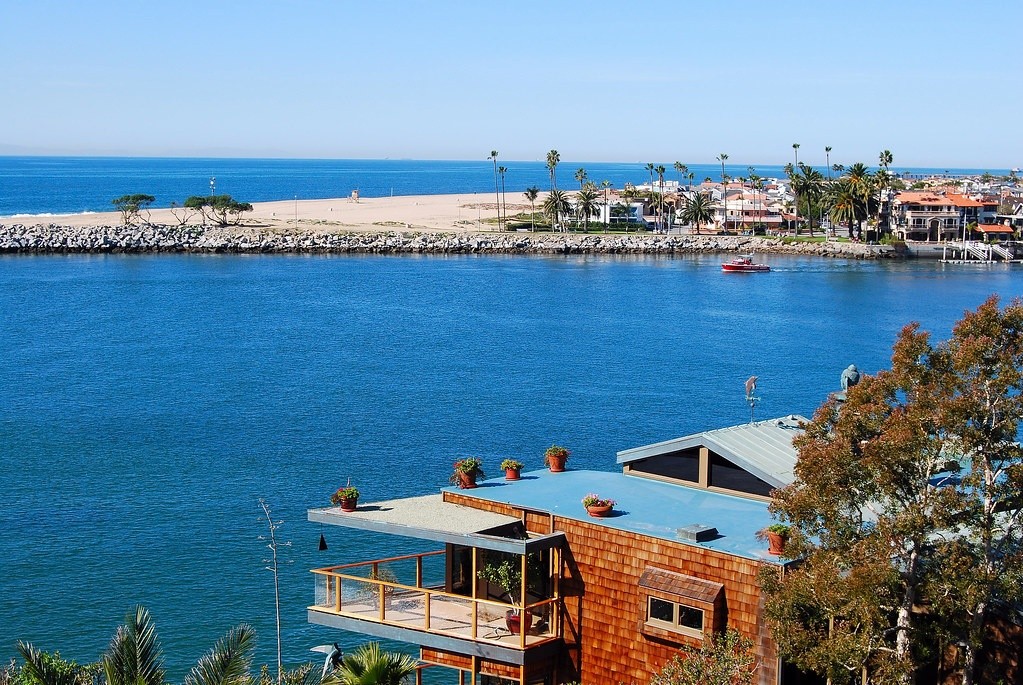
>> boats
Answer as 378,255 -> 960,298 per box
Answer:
721,256 -> 770,273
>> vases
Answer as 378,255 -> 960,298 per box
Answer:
459,471 -> 477,488
506,468 -> 520,479
767,532 -> 789,551
548,456 -> 566,469
588,504 -> 615,516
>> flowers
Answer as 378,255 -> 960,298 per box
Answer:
447,454 -> 487,489
754,523 -> 803,544
542,444 -> 571,466
500,458 -> 525,471
580,493 -> 618,512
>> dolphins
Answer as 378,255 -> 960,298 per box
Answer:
743,376 -> 758,398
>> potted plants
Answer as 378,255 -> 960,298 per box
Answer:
330,480 -> 361,513
364,570 -> 401,612
477,526 -> 543,634
